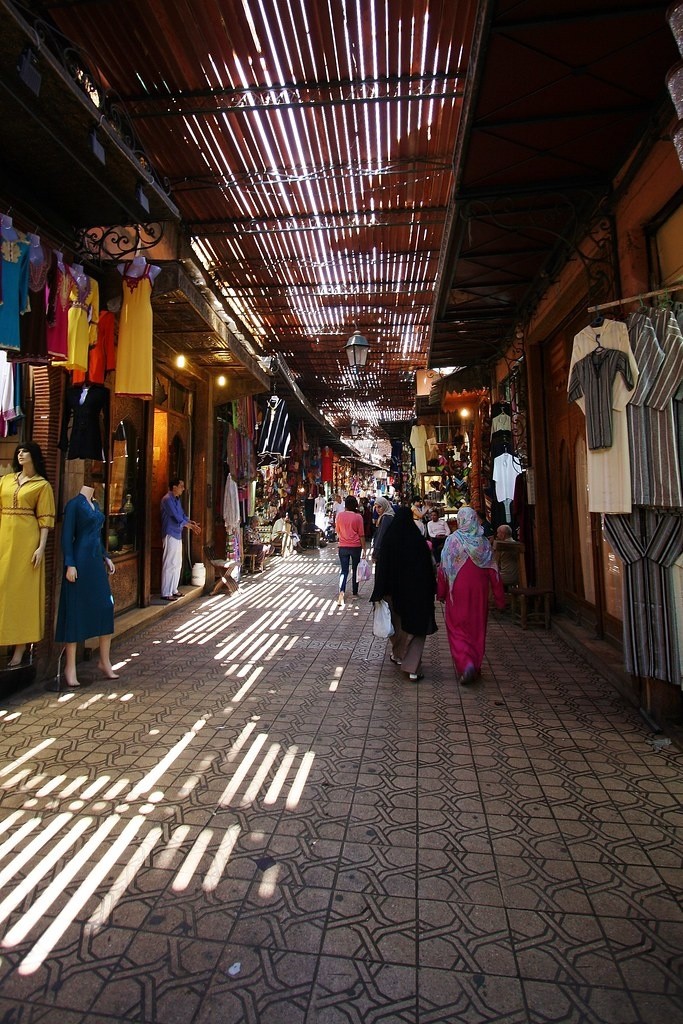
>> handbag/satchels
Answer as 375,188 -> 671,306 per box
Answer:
357,559 -> 371,583
371,600 -> 395,638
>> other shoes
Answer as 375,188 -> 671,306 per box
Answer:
337,592 -> 345,606
353,595 -> 362,600
459,666 -> 475,685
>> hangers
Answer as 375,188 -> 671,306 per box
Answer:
494,286 -> 672,457
268,384 -> 279,405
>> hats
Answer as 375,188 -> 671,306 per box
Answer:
413,495 -> 422,502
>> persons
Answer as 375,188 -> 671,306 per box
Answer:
436,506 -> 505,685
306,492 -> 326,531
428,510 -> 451,567
271,509 -> 307,556
160,478 -> 201,601
0,440 -> 57,669
372,497 -> 395,565
371,506 -> 438,680
488,524 -> 524,610
334,495 -> 366,606
54,484 -> 119,686
244,515 -> 270,572
476,509 -> 494,545
339,491 -> 437,542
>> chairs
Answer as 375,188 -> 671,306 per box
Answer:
203,545 -> 240,597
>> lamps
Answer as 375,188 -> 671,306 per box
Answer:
342,293 -> 371,367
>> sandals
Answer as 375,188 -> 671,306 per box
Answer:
173,592 -> 186,597
389,652 -> 402,665
162,596 -> 177,601
409,673 -> 426,682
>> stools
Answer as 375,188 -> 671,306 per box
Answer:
244,552 -> 264,573
509,587 -> 554,629
270,543 -> 283,556
